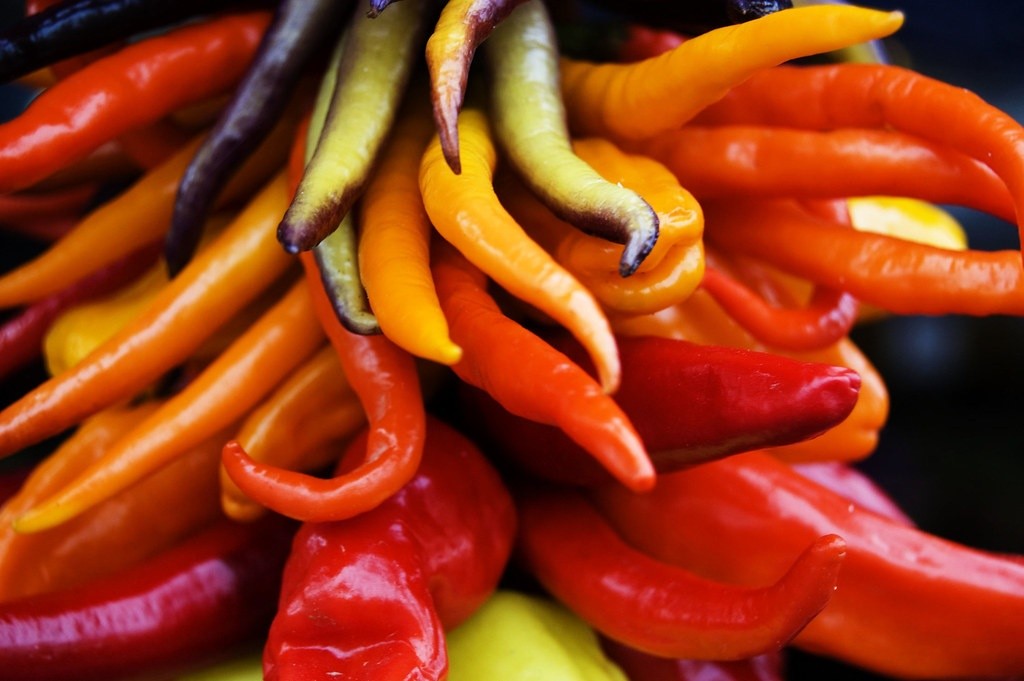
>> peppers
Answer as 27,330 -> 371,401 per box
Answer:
0,0 -> 1024,681
